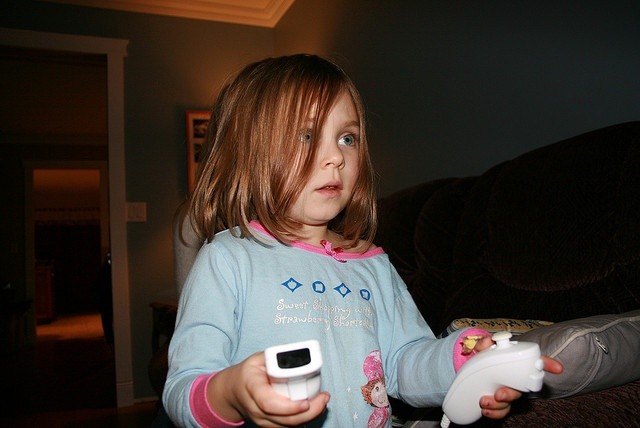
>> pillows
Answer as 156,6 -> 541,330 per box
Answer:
437,317 -> 554,364
512,309 -> 640,400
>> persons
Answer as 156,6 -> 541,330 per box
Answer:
162,53 -> 564,428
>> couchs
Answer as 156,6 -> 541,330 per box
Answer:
71,121 -> 640,428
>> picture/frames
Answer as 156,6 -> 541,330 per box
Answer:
185,109 -> 213,195
